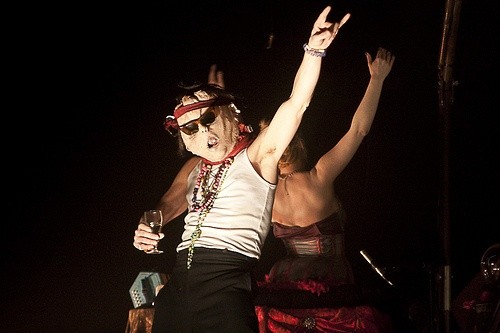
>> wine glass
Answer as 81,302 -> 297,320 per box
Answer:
143,211 -> 164,255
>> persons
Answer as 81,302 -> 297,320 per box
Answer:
256,48 -> 395,333
133,6 -> 351,333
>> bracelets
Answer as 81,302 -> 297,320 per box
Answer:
303,43 -> 326,57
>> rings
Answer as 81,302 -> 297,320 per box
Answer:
138,243 -> 142,250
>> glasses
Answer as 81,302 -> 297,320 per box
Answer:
178,107 -> 216,135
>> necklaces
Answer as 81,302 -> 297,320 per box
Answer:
187,156 -> 234,268
278,170 -> 297,195
